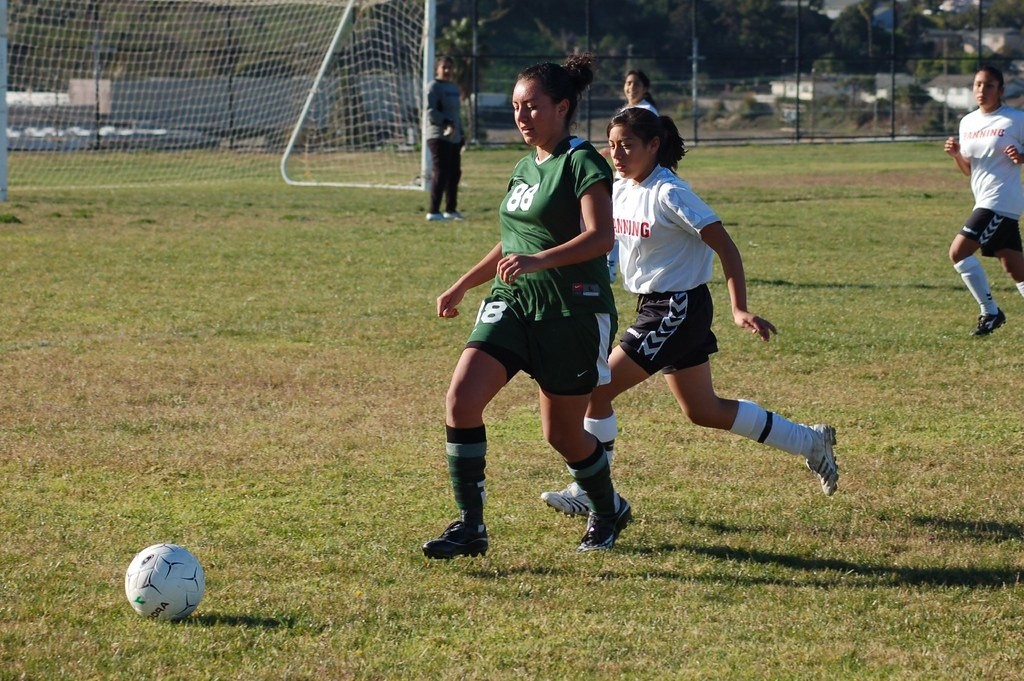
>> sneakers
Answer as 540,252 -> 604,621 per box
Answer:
422,521 -> 489,560
540,482 -> 592,518
576,494 -> 633,553
804,424 -> 839,496
969,307 -> 1006,336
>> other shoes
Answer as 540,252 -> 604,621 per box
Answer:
426,213 -> 447,220
608,265 -> 616,283
441,211 -> 463,219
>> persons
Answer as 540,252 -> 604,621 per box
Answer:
426,55 -> 466,220
540,106 -> 839,517
606,68 -> 659,283
421,53 -> 633,560
945,67 -> 1024,335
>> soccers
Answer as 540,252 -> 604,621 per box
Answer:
124,542 -> 205,622
